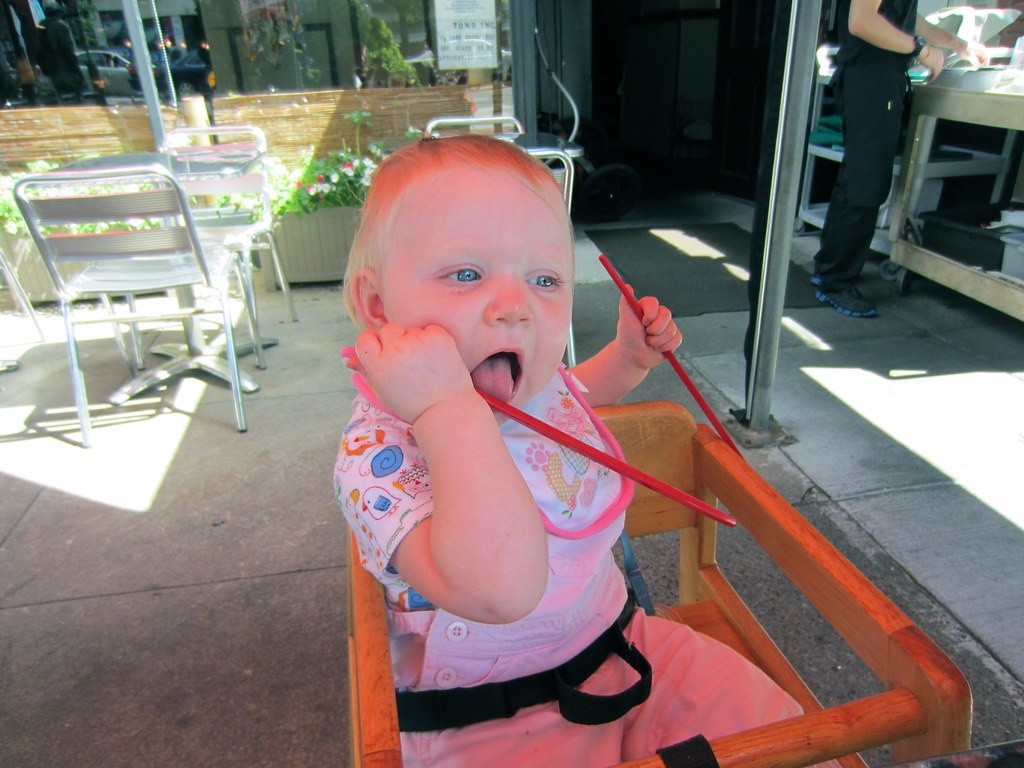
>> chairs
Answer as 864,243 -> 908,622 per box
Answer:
423,115 -> 577,371
14,165 -> 270,451
340,401 -> 975,768
160,123 -> 298,369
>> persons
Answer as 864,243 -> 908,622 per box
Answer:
805,0 -> 990,320
332,133 -> 803,767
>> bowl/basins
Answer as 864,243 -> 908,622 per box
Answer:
938,70 -> 1002,91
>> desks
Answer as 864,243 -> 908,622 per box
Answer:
25,142 -> 269,374
372,131 -> 584,160
794,76 -> 1024,320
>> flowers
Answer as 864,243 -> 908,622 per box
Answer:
0,75 -> 442,239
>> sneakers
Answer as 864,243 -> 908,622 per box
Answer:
810,268 -> 825,286
816,284 -> 877,317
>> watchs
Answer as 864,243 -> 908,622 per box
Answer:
909,34 -> 925,57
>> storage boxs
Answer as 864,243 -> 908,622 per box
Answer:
0,206 -> 363,314
918,200 -> 1024,271
928,68 -> 1005,91
999,233 -> 1024,281
